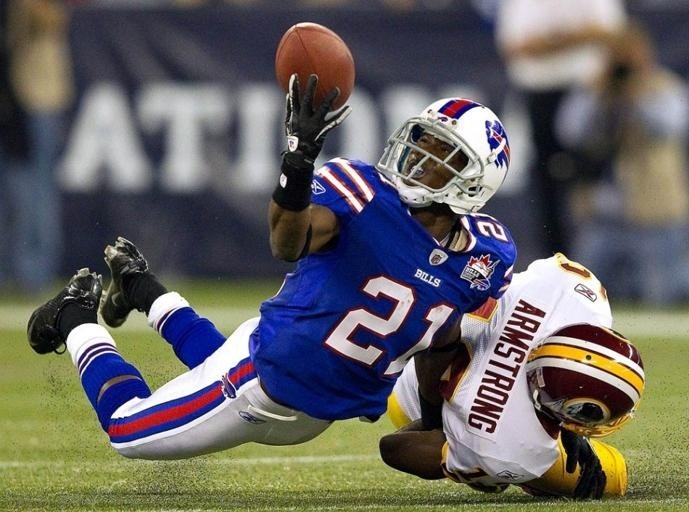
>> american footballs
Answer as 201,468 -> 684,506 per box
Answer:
275,23 -> 355,111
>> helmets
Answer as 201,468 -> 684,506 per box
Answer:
375,96 -> 511,218
525,322 -> 646,439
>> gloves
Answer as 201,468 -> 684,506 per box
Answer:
560,425 -> 608,502
270,72 -> 352,213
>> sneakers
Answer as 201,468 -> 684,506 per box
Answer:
26,267 -> 104,355
100,237 -> 150,329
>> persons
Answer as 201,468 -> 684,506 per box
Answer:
552,17 -> 689,313
493,0 -> 634,254
378,249 -> 648,500
1,0 -> 77,294
26,69 -> 520,463
